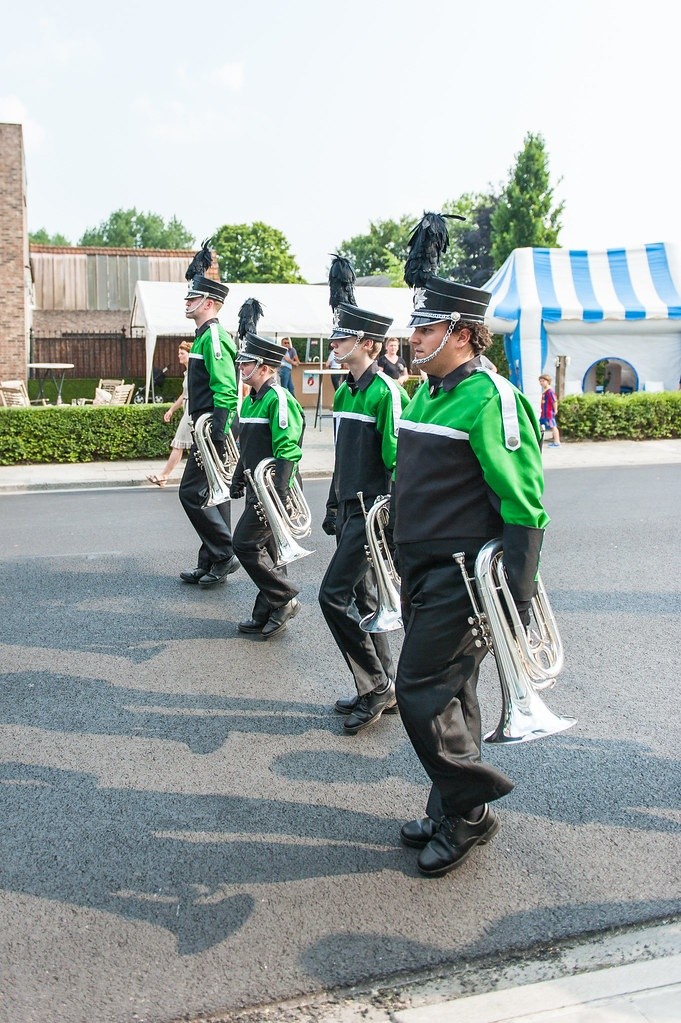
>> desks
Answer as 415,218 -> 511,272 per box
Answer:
28,362 -> 74,405
304,369 -> 351,432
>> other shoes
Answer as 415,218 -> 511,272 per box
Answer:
547,443 -> 560,448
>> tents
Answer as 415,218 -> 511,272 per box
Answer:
477,241 -> 681,431
129,280 -> 424,406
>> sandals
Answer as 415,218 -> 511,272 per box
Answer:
145,475 -> 168,487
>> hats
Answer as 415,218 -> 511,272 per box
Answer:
234,298 -> 288,367
328,254 -> 394,342
184,237 -> 229,305
404,210 -> 493,328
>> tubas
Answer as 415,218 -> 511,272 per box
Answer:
453,534 -> 579,747
243,457 -> 317,571
356,491 -> 405,634
186,411 -> 240,510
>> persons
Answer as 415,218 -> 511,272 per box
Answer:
318,301 -> 410,732
233,331 -> 305,638
178,276 -> 241,586
391,278 -> 549,877
144,338 -> 561,487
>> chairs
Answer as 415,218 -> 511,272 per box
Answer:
0,377 -> 135,409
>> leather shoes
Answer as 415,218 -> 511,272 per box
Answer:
238,597 -> 302,637
180,568 -> 227,584
344,678 -> 398,733
198,554 -> 242,585
401,802 -> 501,878
335,695 -> 398,714
